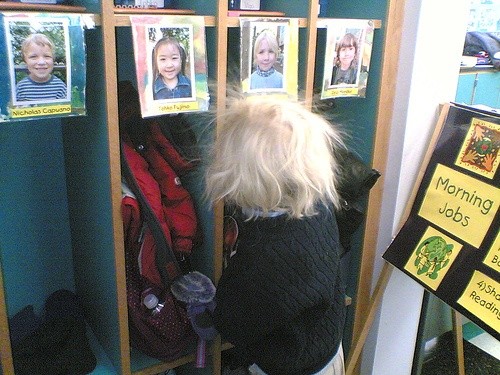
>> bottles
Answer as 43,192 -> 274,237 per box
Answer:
144,294 -> 166,324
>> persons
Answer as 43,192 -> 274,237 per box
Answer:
204,94 -> 347,375
16,34 -> 67,100
152,37 -> 191,100
332,34 -> 357,84
251,30 -> 283,88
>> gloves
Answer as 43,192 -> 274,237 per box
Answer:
191,298 -> 218,343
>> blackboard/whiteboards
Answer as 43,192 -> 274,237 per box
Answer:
379,100 -> 500,341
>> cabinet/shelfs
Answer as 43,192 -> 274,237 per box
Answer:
455,66 -> 500,113
0,0 -> 406,375
